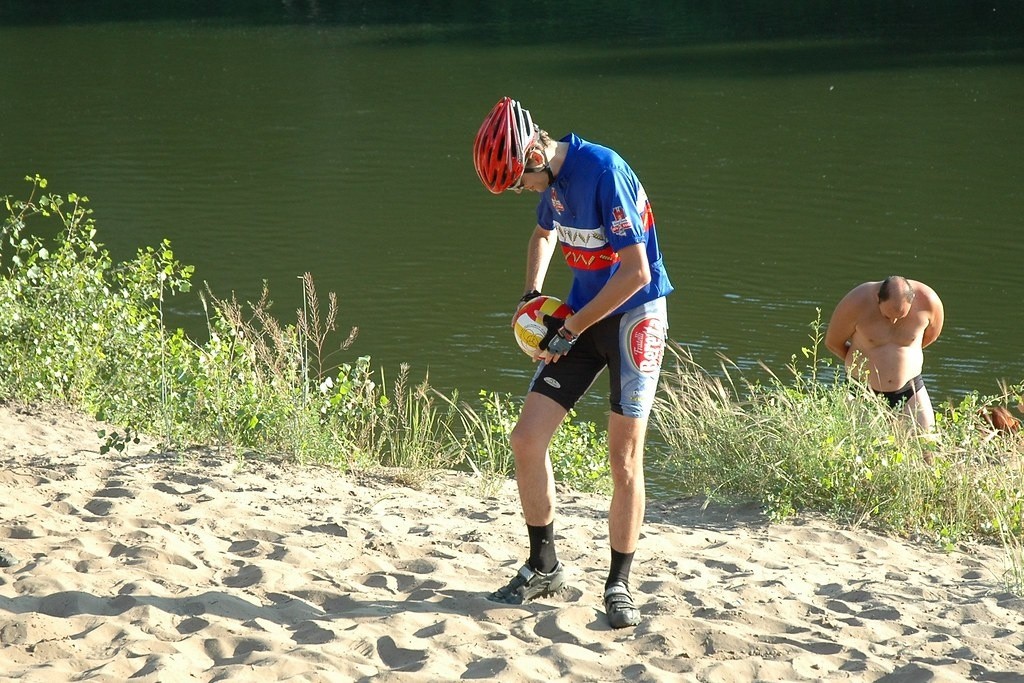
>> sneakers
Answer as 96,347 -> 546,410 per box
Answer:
603,580 -> 641,629
485,559 -> 564,604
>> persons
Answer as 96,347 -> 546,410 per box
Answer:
473,96 -> 674,628
825,276 -> 945,467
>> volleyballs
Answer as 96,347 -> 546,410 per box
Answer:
513,294 -> 578,362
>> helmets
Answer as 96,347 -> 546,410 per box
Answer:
472,95 -> 538,195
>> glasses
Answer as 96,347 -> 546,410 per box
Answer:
505,150 -> 534,191
